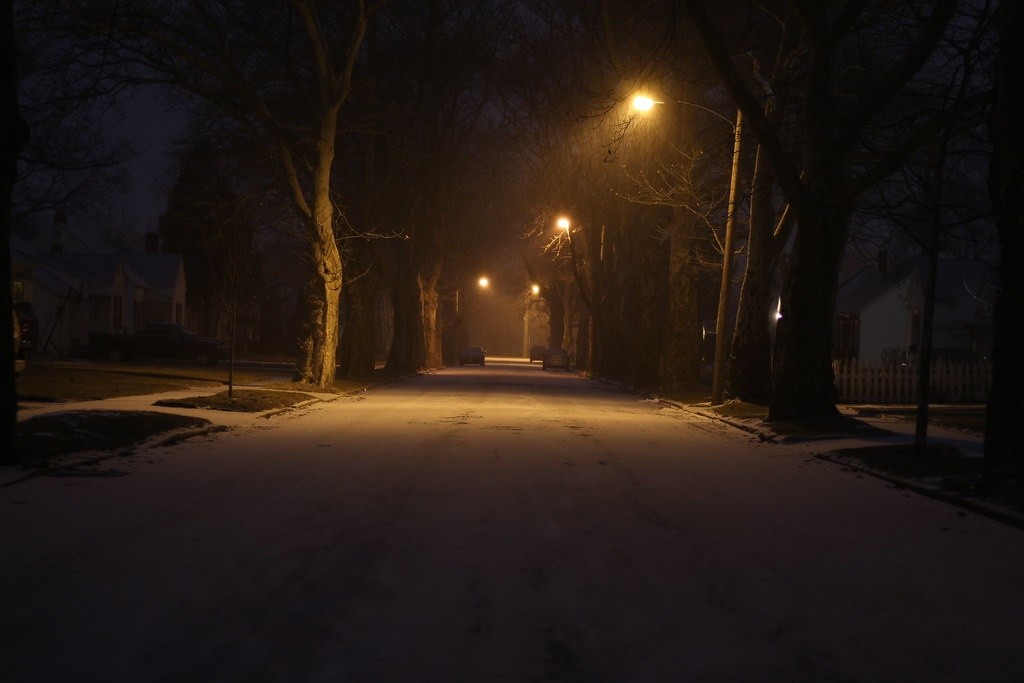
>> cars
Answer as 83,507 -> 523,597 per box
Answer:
457,344 -> 487,366
529,344 -> 546,364
542,347 -> 570,371
84,319 -> 225,366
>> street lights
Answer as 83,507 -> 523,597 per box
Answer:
632,93 -> 750,409
